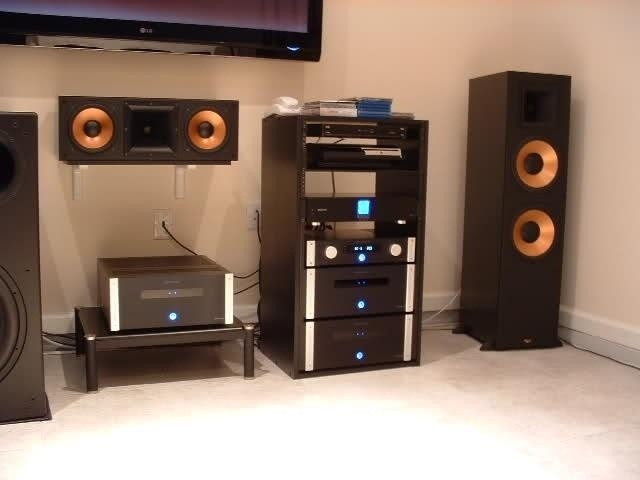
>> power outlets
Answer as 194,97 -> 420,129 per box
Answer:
152,209 -> 175,240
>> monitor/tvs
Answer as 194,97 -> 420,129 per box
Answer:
0,0 -> 323,61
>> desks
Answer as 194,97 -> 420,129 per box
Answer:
75,306 -> 255,391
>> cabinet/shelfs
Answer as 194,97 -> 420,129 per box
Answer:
262,114 -> 430,379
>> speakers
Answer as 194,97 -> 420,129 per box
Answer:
0,112 -> 52,425
59,96 -> 239,165
451,70 -> 571,351
